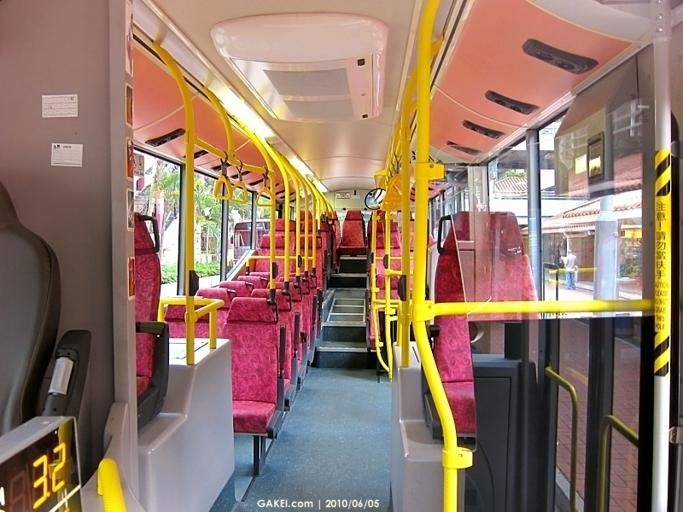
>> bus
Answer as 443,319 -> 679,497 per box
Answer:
231,219 -> 270,263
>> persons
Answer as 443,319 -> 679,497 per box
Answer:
565,250 -> 578,290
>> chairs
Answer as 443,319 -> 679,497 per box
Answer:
135,208 -> 549,478
2,185 -> 92,444
